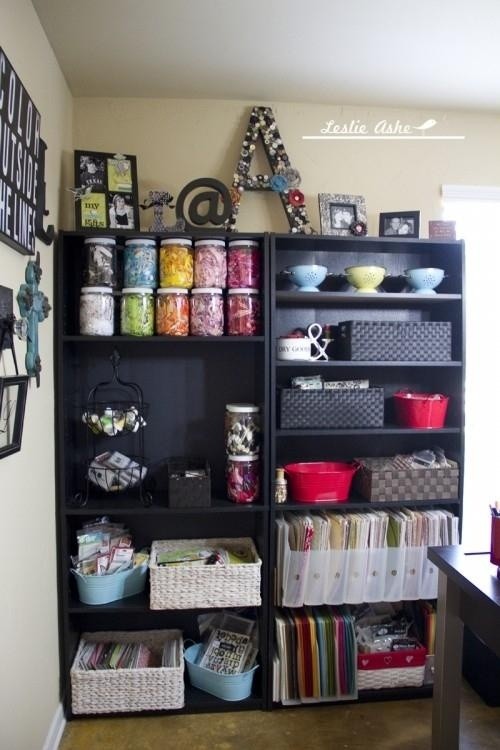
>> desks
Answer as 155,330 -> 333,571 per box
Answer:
428,545 -> 500,750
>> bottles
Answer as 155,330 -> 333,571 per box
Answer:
323,322 -> 332,339
275,468 -> 288,504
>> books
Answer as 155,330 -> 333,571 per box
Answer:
196,613 -> 258,677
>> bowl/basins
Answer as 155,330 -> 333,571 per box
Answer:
284,461 -> 362,503
393,388 -> 449,430
279,263 -> 334,290
339,264 -> 393,291
397,265 -> 452,292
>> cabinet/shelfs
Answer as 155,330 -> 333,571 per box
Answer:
269,234 -> 469,707
55,228 -> 268,718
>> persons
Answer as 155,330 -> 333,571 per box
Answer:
384,217 -> 402,235
398,222 -> 412,235
333,207 -> 345,230
342,207 -> 355,229
108,193 -> 136,230
80,158 -> 106,190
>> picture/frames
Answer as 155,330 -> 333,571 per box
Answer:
318,192 -> 367,236
379,210 -> 420,238
74,149 -> 140,234
0,374 -> 30,459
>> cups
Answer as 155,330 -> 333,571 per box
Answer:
274,323 -> 332,361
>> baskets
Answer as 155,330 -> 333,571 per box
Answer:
338,320 -> 453,361
81,402 -> 150,441
81,455 -> 154,499
149,537 -> 262,611
358,664 -> 426,690
357,456 -> 459,504
70,629 -> 186,715
280,385 -> 386,430
